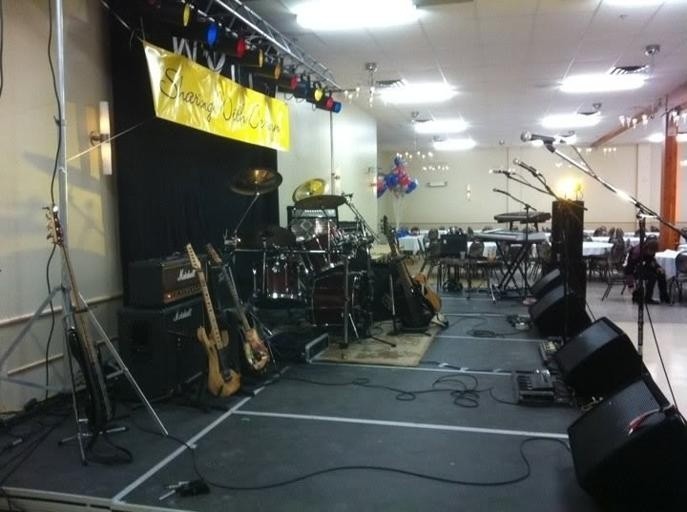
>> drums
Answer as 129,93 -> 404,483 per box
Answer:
313,272 -> 363,336
295,234 -> 345,278
251,258 -> 299,309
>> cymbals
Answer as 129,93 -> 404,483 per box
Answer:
247,225 -> 295,245
292,179 -> 325,203
224,166 -> 282,196
294,195 -> 348,210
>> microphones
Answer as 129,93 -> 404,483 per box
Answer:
489,168 -> 511,176
513,158 -> 539,176
493,187 -> 514,196
520,131 -> 567,144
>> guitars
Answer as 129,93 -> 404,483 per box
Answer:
186,243 -> 241,397
204,243 -> 270,372
380,215 -> 441,327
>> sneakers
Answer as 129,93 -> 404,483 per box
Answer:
645,297 -> 670,304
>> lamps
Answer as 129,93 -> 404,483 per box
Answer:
145,0 -> 341,112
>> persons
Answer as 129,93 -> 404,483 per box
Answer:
626,233 -> 671,305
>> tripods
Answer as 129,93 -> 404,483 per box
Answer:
241,203 -> 394,347
0,1 -> 169,462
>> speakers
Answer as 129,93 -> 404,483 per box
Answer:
566,374 -> 687,512
528,266 -> 560,297
117,295 -> 205,397
551,200 -> 584,298
553,316 -> 646,389
528,282 -> 586,336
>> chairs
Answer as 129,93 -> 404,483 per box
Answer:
395,227 -> 687,306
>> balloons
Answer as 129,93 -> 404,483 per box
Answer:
376,154 -> 419,200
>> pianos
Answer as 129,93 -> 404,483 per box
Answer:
472,228 -> 545,242
495,212 -> 551,223
